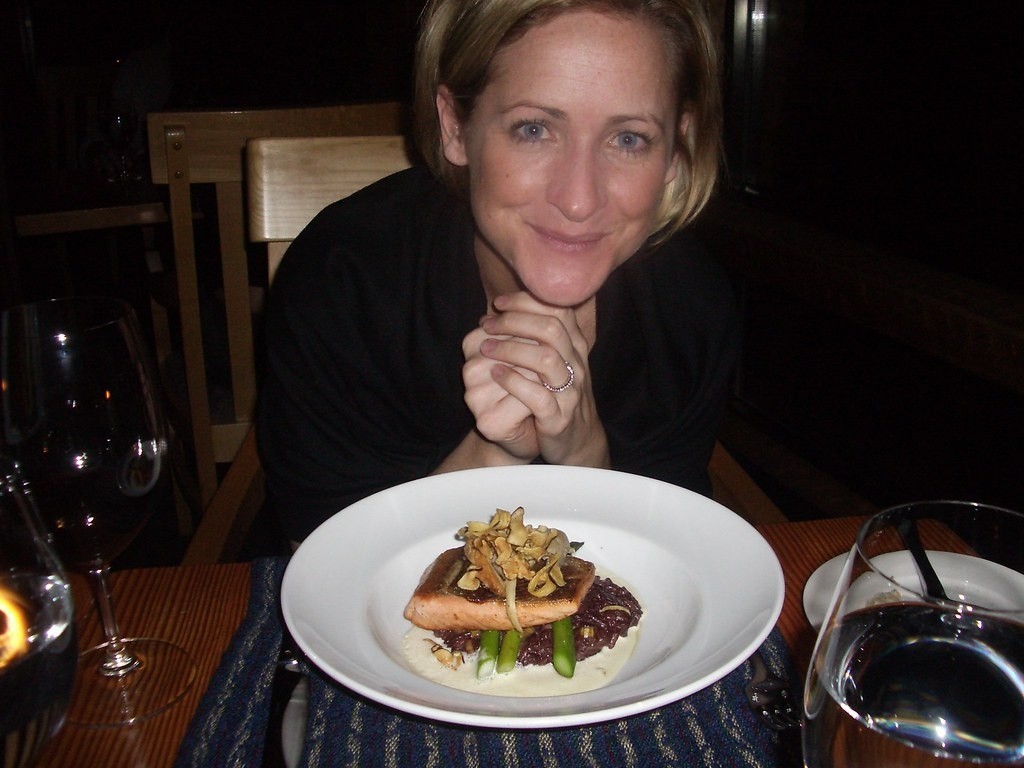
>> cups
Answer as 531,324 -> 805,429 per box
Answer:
0,461 -> 86,768
800,498 -> 1024,768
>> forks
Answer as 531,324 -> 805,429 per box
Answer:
742,650 -> 808,731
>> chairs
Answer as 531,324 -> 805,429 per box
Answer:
149,101 -> 421,512
180,136 -> 789,566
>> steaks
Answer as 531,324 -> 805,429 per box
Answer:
404,546 -> 595,629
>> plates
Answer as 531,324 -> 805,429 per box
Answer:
280,464 -> 786,730
803,549 -> 1024,636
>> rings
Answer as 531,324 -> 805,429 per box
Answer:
543,362 -> 574,392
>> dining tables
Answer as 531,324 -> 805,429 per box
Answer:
0,515 -> 978,768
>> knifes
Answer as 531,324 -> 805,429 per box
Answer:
282,677 -> 309,768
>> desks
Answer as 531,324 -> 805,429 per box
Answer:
12,188 -> 208,538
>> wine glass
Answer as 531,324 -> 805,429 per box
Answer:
0,298 -> 200,730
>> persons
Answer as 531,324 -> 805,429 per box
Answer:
251,0 -> 736,556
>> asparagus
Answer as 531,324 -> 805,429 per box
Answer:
478,633 -> 496,678
553,619 -> 576,677
499,632 -> 520,671
